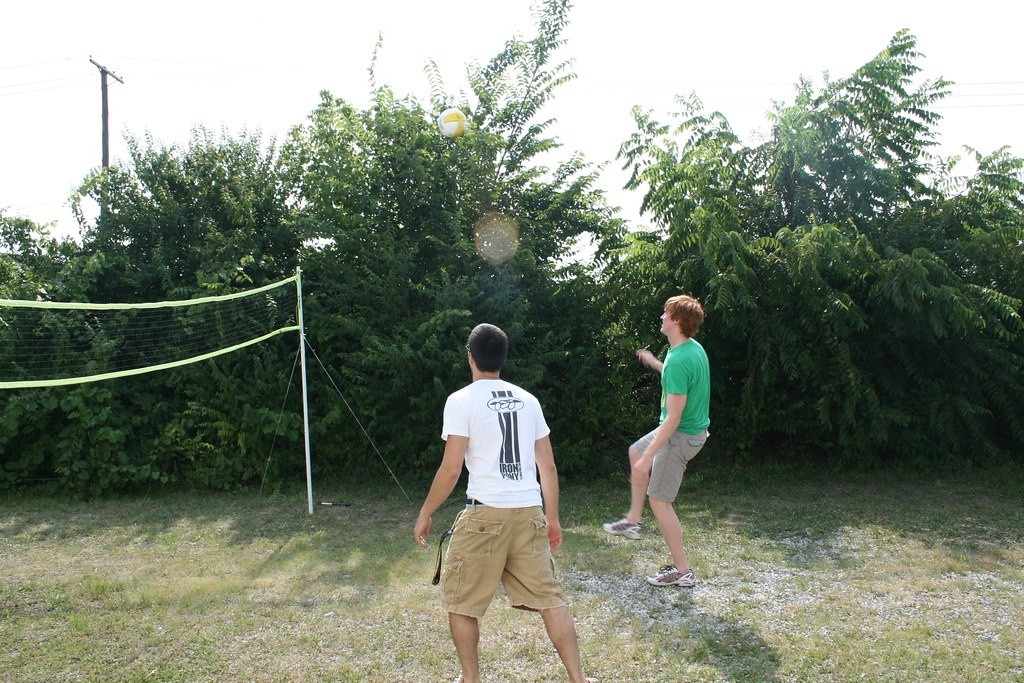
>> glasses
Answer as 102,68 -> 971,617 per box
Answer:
465,344 -> 469,351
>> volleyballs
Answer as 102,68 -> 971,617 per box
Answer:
438,108 -> 468,138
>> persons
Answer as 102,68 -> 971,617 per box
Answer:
602,295 -> 711,586
416,324 -> 602,683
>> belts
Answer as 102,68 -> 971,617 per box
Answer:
466,499 -> 483,506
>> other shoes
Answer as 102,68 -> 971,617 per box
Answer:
453,669 -> 463,683
585,677 -> 599,683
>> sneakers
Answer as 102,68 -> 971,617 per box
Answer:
647,565 -> 696,586
604,519 -> 644,540
